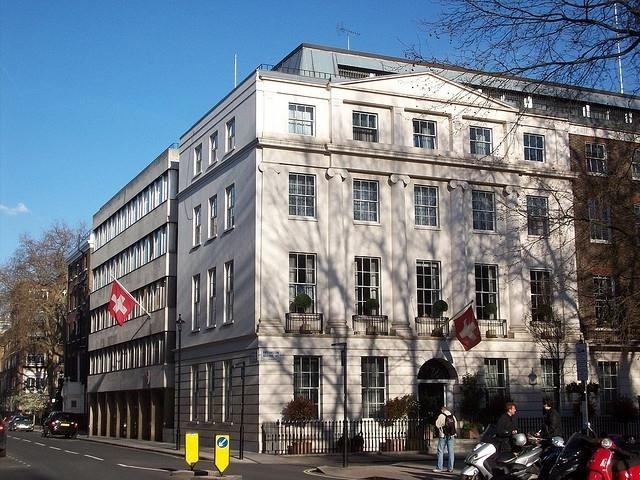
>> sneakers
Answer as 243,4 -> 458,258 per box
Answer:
432,469 -> 454,473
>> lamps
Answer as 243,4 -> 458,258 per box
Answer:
528,367 -> 538,390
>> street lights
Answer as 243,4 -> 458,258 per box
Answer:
174,314 -> 186,450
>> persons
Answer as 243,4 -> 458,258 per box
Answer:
535,397 -> 563,438
495,402 -> 519,453
432,405 -> 458,472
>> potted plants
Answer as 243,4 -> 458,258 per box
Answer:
432,299 -> 448,338
295,294 -> 313,334
538,306 -> 554,339
462,423 -> 482,439
338,434 -> 364,454
279,397 -> 315,454
371,394 -> 422,453
364,298 -> 379,334
485,303 -> 497,338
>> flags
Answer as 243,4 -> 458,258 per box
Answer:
454,305 -> 481,351
108,280 -> 136,326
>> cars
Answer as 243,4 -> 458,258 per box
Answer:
0,415 -> 7,457
9,415 -> 34,432
42,411 -> 79,438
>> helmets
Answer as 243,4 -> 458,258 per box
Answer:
511,432 -> 528,446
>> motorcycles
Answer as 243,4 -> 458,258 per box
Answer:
462,422 -> 640,479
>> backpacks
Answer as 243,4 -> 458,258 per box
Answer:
439,412 -> 457,437
